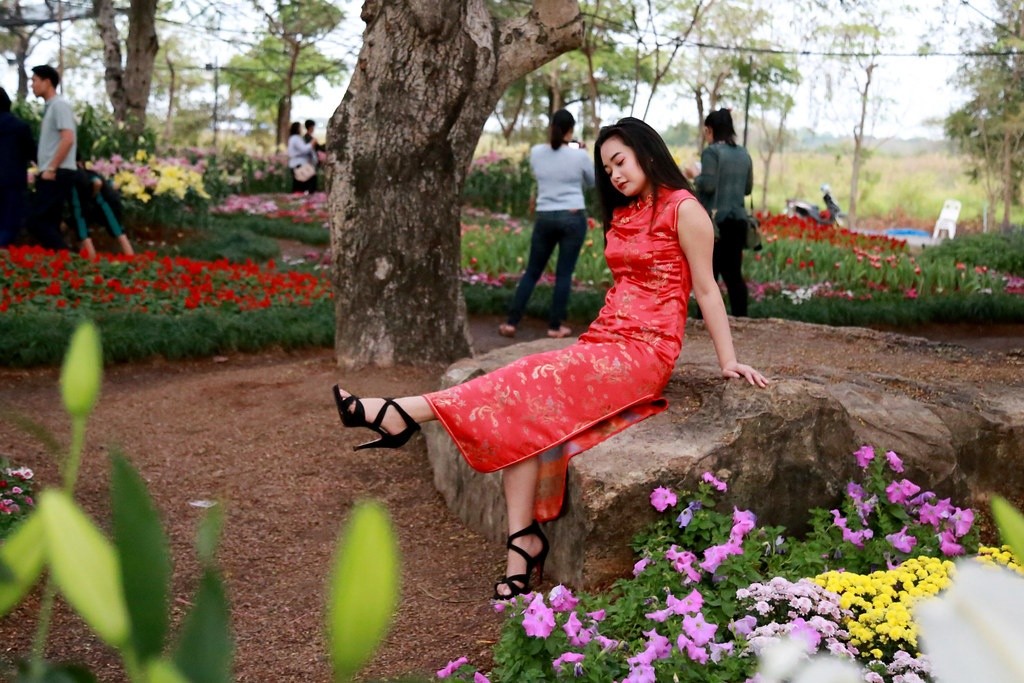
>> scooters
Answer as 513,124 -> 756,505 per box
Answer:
783,185 -> 850,232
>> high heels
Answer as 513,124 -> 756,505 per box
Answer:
332,383 -> 422,451
493,519 -> 549,601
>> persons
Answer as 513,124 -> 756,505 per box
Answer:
497,109 -> 595,339
287,119 -> 326,195
332,117 -> 771,600
0,65 -> 135,261
684,107 -> 754,320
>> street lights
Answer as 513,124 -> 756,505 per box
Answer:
205,57 -> 219,148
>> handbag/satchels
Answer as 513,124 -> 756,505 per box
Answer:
711,217 -> 719,242
294,161 -> 317,182
745,215 -> 762,251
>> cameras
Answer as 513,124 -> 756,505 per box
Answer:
568,141 -> 582,150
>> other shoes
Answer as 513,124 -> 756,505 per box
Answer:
548,327 -> 572,338
498,323 -> 515,336
